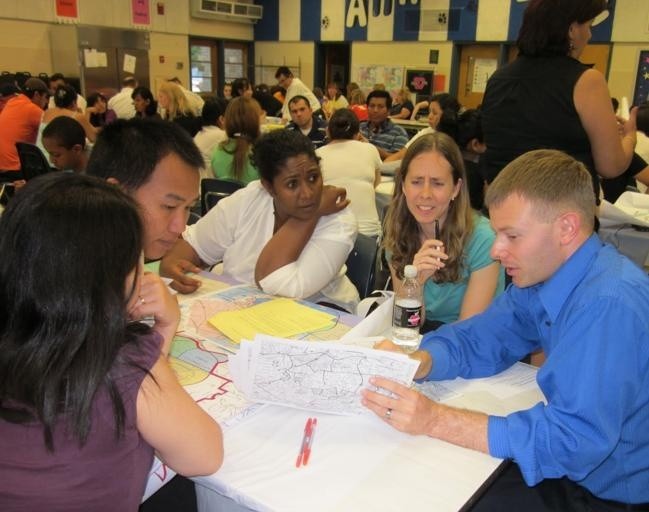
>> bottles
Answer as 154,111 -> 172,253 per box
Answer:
390,265 -> 420,349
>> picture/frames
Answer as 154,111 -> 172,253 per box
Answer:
420,373 -> 422,375
244,335 -> 419,416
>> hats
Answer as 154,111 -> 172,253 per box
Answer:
24,78 -> 53,96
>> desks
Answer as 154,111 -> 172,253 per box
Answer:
390,116 -> 430,134
375,172 -> 396,211
142,252 -> 546,512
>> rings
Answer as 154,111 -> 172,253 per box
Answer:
139,295 -> 145,304
385,409 -> 392,419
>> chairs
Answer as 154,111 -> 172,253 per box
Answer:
200,177 -> 247,218
0,141 -> 55,179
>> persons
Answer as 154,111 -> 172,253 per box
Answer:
388,87 -> 413,119
159,82 -> 196,135
192,97 -> 229,202
48,72 -> 88,118
438,110 -> 487,212
81,115 -> 206,261
359,148 -> 649,512
379,131 -> 506,323
231,77 -> 252,99
157,128 -> 360,315
346,83 -> 361,104
0,76 -> 52,184
275,66 -> 322,127
223,82 -> 231,97
0,172 -> 224,512
601,148 -> 649,204
349,89 -> 368,123
107,76 -> 139,120
35,84 -> 101,171
0,78 -> 17,111
86,92 -> 116,128
283,95 -> 329,150
323,82 -> 349,115
357,90 -> 409,160
482,0 -> 639,220
382,92 -> 461,163
314,106 -> 383,301
40,115 -> 95,177
131,86 -> 161,121
410,94 -> 431,121
207,96 -> 263,187
312,86 -> 325,108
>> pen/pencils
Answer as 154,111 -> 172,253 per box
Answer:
296,417 -> 311,468
302,417 -> 318,467
434,219 -> 442,268
